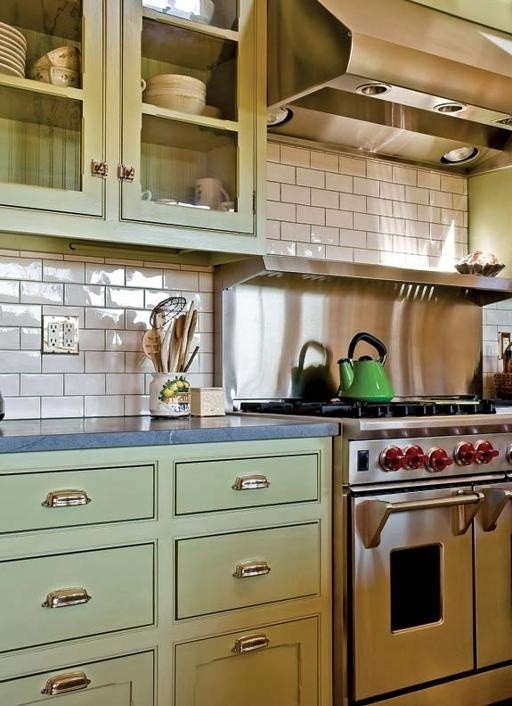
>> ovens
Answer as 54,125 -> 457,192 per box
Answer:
333,473 -> 512,706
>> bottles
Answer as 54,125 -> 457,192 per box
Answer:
148,371 -> 192,417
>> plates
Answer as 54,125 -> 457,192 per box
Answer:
454,262 -> 505,277
0,21 -> 28,78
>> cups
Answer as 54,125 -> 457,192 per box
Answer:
194,176 -> 231,210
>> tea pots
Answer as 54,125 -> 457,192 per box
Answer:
289,338 -> 337,401
335,333 -> 394,403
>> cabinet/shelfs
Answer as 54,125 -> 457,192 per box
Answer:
0,433 -> 339,706
0,0 -> 274,265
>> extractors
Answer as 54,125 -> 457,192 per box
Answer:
205,0 -> 511,178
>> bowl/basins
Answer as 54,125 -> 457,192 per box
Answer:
203,104 -> 224,118
31,45 -> 82,88
145,74 -> 208,117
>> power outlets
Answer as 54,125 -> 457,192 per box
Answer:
42,314 -> 81,355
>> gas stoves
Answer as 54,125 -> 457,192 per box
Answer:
234,397 -> 511,485
